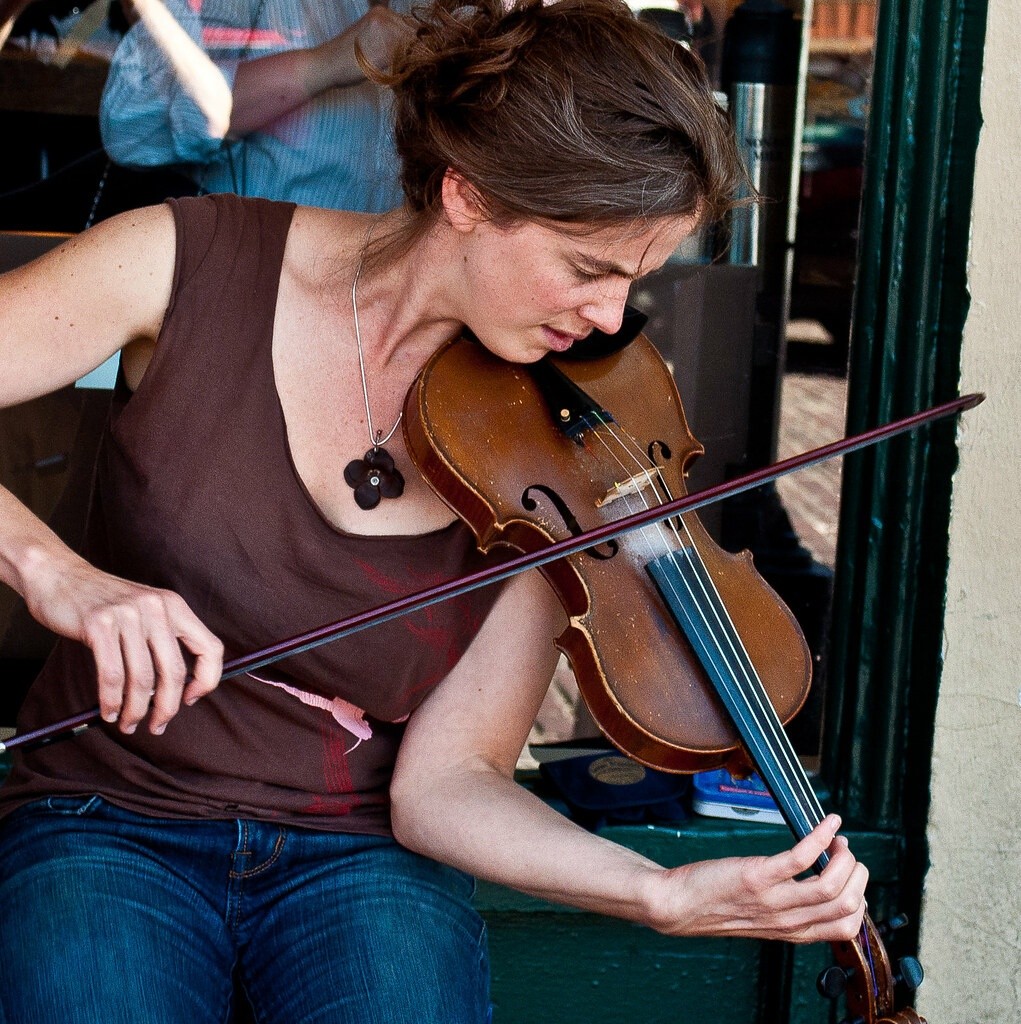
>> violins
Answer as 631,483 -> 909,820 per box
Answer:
401,319 -> 929,1024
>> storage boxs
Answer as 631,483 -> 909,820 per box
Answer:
690,768 -> 787,826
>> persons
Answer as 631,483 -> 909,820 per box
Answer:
101,0 -> 441,218
2,2 -> 871,1023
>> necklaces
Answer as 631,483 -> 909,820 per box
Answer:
343,206 -> 438,512
